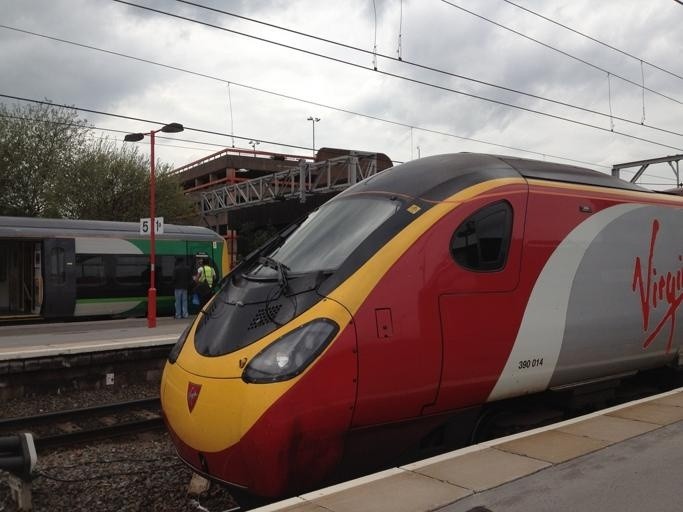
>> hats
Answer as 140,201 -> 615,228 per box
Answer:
195,252 -> 209,258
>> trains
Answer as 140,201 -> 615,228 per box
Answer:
0,216 -> 230,329
155,151 -> 682,506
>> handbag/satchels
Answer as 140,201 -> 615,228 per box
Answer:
194,279 -> 212,300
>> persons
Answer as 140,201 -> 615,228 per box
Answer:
171,251 -> 219,319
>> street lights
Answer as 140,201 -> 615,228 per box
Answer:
306,116 -> 320,161
248,141 -> 260,157
123,123 -> 185,330
